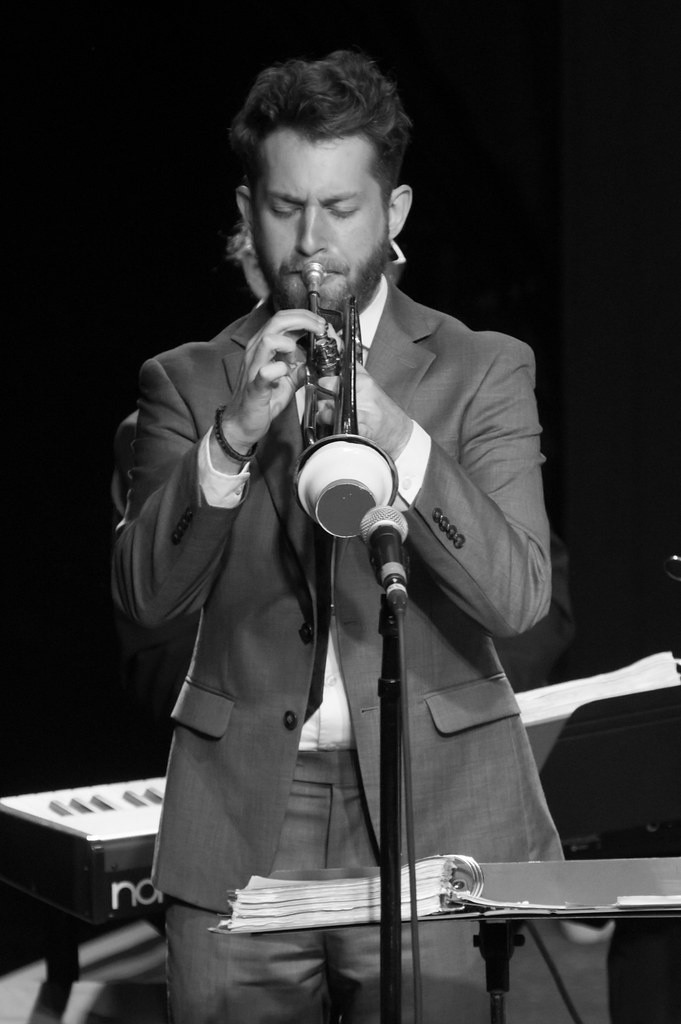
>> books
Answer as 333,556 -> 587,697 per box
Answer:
210,854 -> 681,935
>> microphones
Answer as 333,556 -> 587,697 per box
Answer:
360,505 -> 409,610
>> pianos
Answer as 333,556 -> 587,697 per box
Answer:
1,775 -> 170,923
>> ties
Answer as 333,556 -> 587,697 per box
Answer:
303,349 -> 333,725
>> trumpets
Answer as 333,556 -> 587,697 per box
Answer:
292,261 -> 401,539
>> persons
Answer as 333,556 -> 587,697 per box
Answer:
114,54 -> 568,1024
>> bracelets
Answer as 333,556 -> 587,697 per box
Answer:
215,405 -> 256,461
